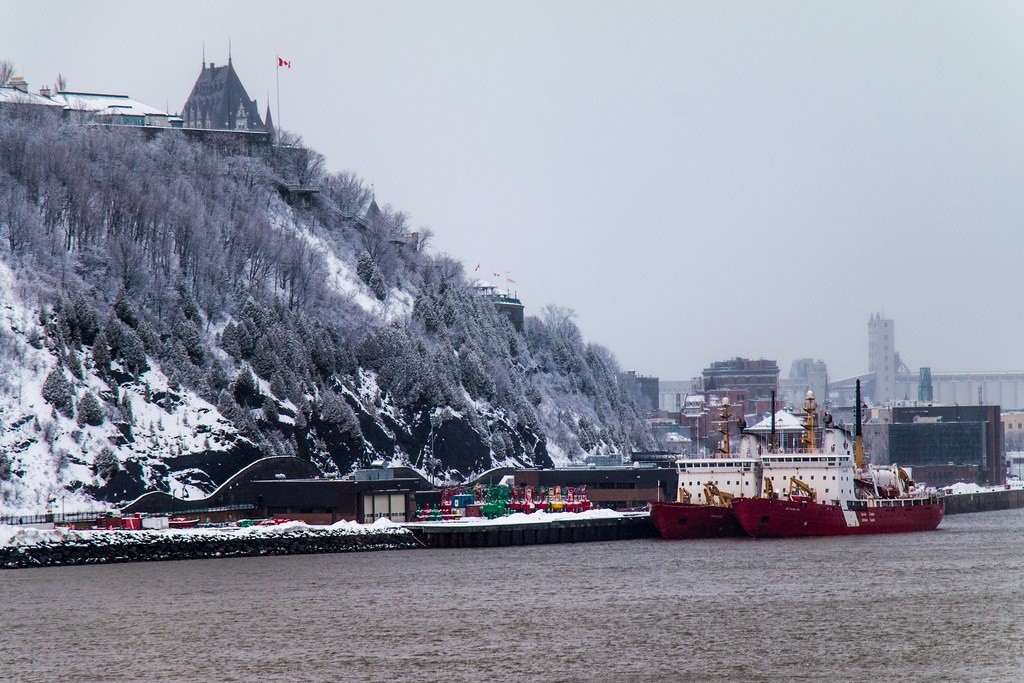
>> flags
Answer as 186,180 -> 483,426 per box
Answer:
278,57 -> 291,69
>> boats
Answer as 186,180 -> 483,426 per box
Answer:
646,452 -> 947,539
